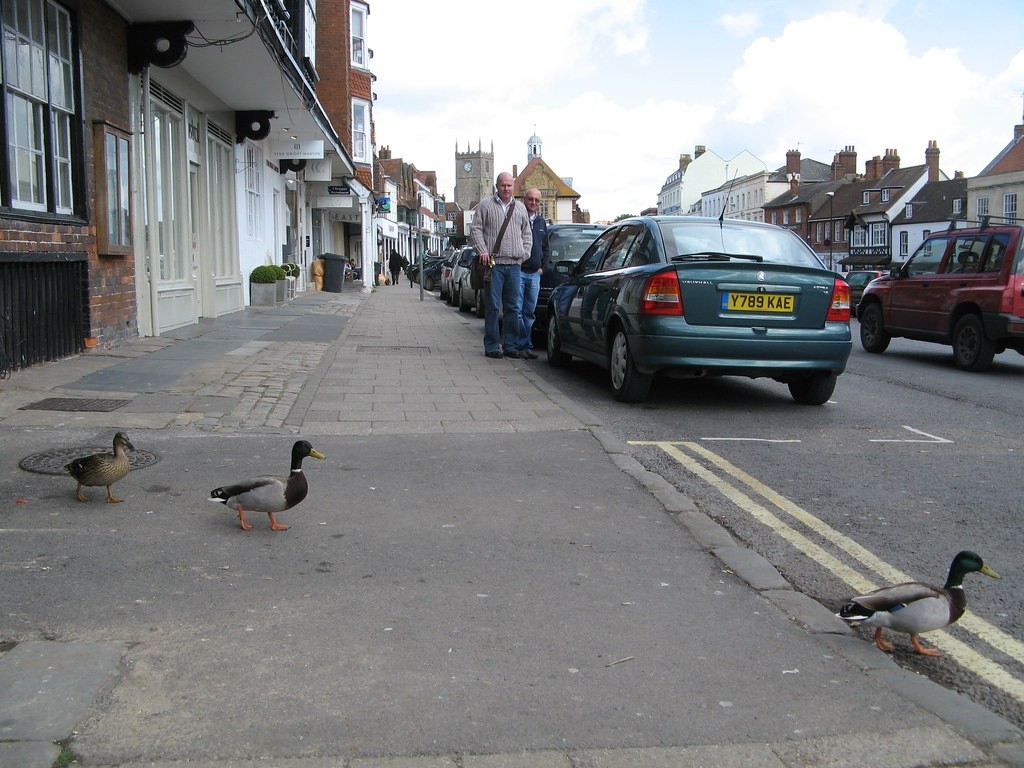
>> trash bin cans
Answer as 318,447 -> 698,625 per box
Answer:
374,262 -> 382,286
323,253 -> 347,292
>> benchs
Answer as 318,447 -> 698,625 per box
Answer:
345,268 -> 353,282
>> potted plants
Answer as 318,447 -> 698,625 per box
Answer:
271,265 -> 287,300
288,262 -> 301,299
250,265 -> 277,306
280,264 -> 294,300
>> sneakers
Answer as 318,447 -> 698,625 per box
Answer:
503,350 -> 520,358
485,351 -> 503,358
519,350 -> 539,359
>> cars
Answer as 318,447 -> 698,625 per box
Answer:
441,247 -> 487,318
547,215 -> 852,403
406,256 -> 446,291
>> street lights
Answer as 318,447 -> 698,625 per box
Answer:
825,192 -> 835,270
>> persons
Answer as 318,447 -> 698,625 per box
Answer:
344,257 -> 359,280
558,229 -> 641,343
390,247 -> 409,285
470,172 -> 552,359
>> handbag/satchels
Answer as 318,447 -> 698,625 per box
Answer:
470,256 -> 495,289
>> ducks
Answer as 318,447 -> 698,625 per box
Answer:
64,431 -> 137,504
834,550 -> 1002,656
207,440 -> 327,532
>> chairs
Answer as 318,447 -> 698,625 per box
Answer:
951,252 -> 979,273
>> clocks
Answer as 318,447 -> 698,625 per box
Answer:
464,162 -> 471,172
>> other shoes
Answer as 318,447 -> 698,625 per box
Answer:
393,280 -> 395,285
396,279 -> 398,284
356,279 -> 362,281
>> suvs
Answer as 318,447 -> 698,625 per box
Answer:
531,223 -> 611,348
845,271 -> 889,318
855,214 -> 1024,371
